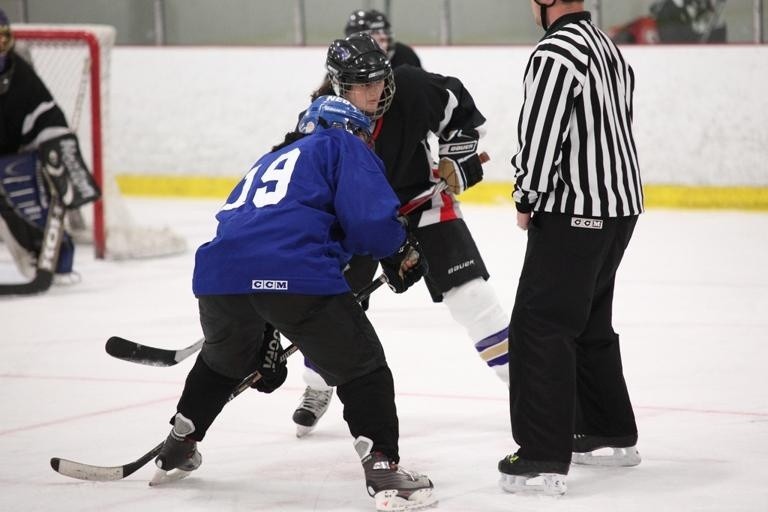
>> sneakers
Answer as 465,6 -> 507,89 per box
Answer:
152,429 -> 203,472
498,452 -> 572,477
361,448 -> 434,494
568,429 -> 638,452
292,386 -> 333,428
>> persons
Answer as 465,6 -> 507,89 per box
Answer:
0,9 -> 100,285
290,34 -> 486,428
345,7 -> 423,74
656,0 -> 727,45
156,94 -> 433,502
498,0 -> 644,475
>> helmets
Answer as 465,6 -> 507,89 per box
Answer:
325,32 -> 398,117
296,96 -> 373,143
343,9 -> 398,60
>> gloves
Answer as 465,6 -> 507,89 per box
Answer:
253,332 -> 291,393
439,151 -> 483,196
377,238 -> 429,294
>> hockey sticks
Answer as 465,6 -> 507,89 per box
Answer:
105,149 -> 490,366
50,273 -> 387,483
0,55 -> 91,296
